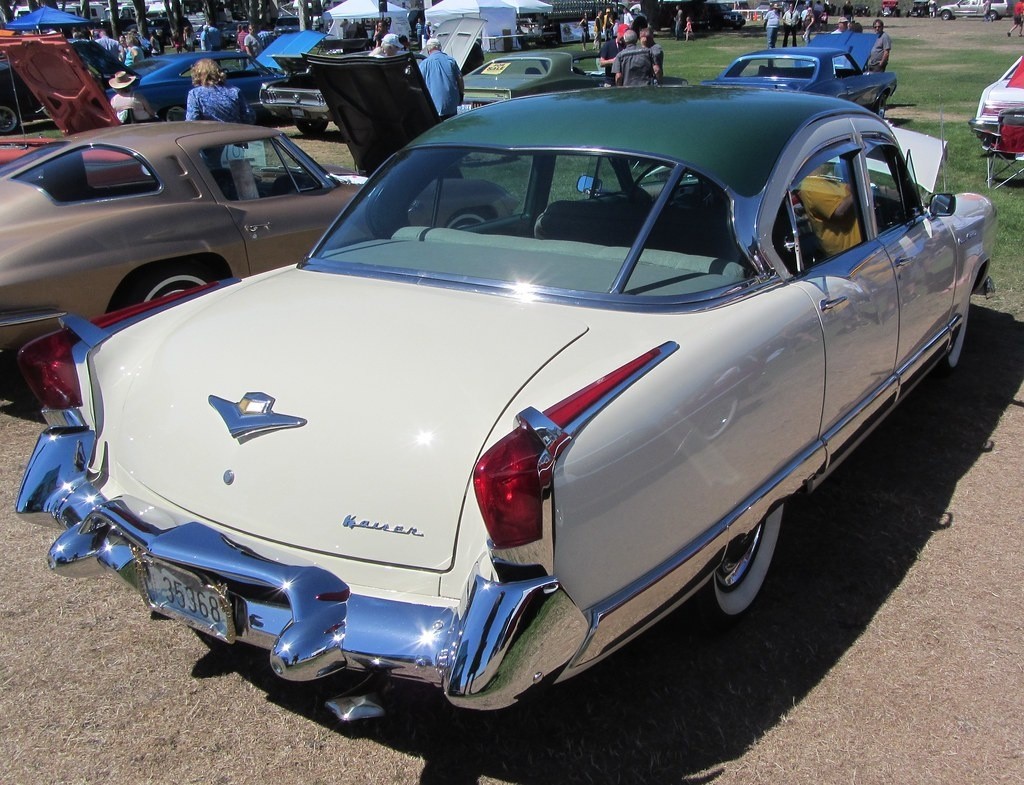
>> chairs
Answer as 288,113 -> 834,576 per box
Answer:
973,108 -> 1024,190
525,68 -> 541,74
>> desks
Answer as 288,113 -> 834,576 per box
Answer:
481,32 -> 557,53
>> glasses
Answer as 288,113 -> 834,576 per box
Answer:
873,26 -> 882,29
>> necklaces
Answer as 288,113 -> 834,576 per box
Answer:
983,0 -> 994,21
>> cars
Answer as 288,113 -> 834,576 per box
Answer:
853,5 -> 871,17
69,30 -> 335,122
259,18 -> 489,135
1,33 -> 156,191
125,18 -> 194,42
755,0 -> 788,19
195,22 -> 250,44
460,50 -> 689,115
12,91 -> 998,725
585,2 -> 647,38
0,39 -> 113,135
105,5 -> 206,31
0,118 -> 521,348
699,2 -> 746,30
966,54 -> 1024,159
700,34 -> 899,118
274,15 -> 301,37
877,0 -> 901,17
906,1 -> 937,18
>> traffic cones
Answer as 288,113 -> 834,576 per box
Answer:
753,12 -> 757,21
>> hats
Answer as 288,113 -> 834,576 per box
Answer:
108,70 -> 137,89
606,8 -> 610,13
617,24 -> 629,37
772,4 -> 780,9
380,33 -> 405,49
203,25 -> 210,30
838,16 -> 849,23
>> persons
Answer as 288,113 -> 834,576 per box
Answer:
419,38 -> 464,122
72,20 -> 274,70
673,5 -> 695,42
368,18 -> 434,59
109,71 -> 160,123
763,0 -> 864,49
341,18 -> 353,39
185,59 -> 256,124
580,7 -> 663,88
798,159 -> 861,255
928,0 -> 938,17
868,20 -> 892,74
1007,0 -> 1024,37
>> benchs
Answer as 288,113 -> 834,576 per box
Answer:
391,227 -> 744,281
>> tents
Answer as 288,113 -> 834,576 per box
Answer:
3,4 -> 91,38
321,0 -> 554,54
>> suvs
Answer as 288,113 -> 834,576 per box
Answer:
938,0 -> 1012,22
